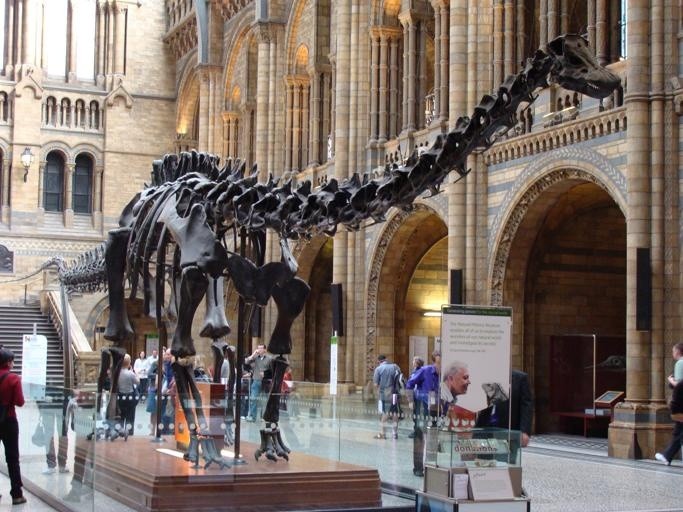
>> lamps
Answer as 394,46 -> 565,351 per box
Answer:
20,148 -> 34,183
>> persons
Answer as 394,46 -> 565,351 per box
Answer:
438,360 -> 471,417
407,356 -> 423,438
61,389 -> 93,501
495,369 -> 532,464
668,379 -> 683,423
0,348 -> 26,505
96,346 -> 293,434
371,355 -> 403,439
405,349 -> 439,476
654,341 -> 682,465
29,383 -> 70,474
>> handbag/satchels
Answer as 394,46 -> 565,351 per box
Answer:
32,423 -> 45,447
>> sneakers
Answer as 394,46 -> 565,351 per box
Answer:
12,496 -> 27,505
42,467 -> 57,475
58,463 -> 69,473
655,452 -> 671,466
63,491 -> 81,501
408,431 -> 415,438
241,415 -> 256,422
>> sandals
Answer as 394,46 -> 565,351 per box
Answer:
392,432 -> 398,440
373,432 -> 387,439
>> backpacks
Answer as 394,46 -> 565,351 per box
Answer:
394,369 -> 407,395
